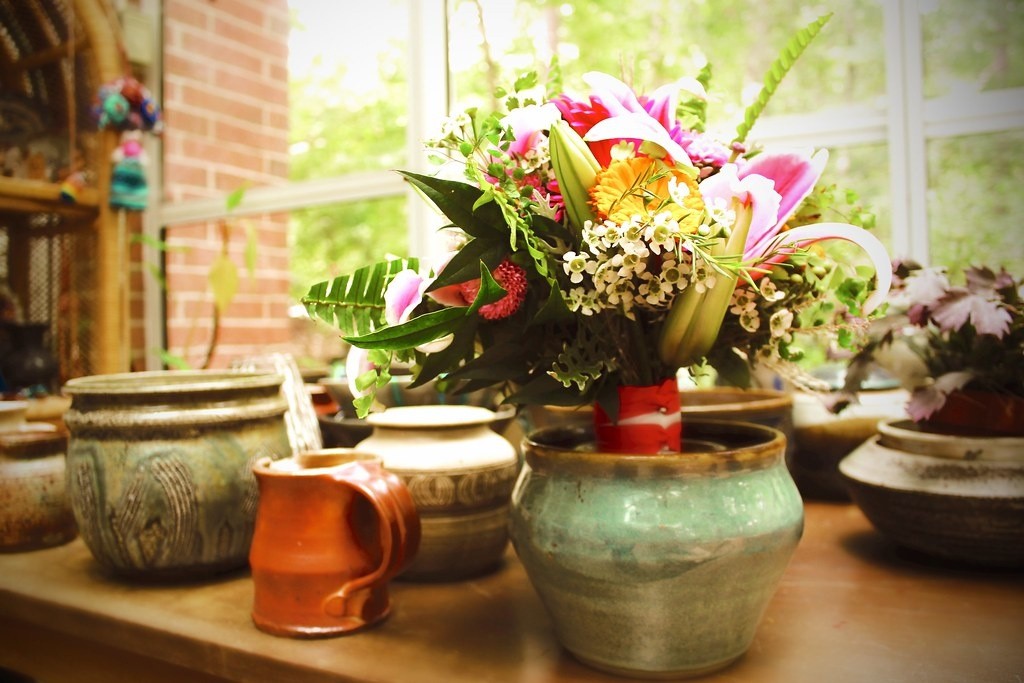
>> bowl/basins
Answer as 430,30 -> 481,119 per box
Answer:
62,370 -> 292,579
318,378 -> 517,450
677,388 -> 796,478
0,429 -> 79,553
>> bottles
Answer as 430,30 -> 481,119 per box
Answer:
0,401 -> 27,430
355,404 -> 518,581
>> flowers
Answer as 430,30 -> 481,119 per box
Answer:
301,10 -> 892,434
829,260 -> 1024,422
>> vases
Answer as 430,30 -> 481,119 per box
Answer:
311,376 -> 525,472
351,404 -> 519,581
0,401 -> 80,556
840,419 -> 1024,568
62,369 -> 292,586
506,417 -> 805,678
540,388 -> 792,463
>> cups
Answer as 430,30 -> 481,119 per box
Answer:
250,449 -> 421,637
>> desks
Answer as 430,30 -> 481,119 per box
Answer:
0,507 -> 1024,683
